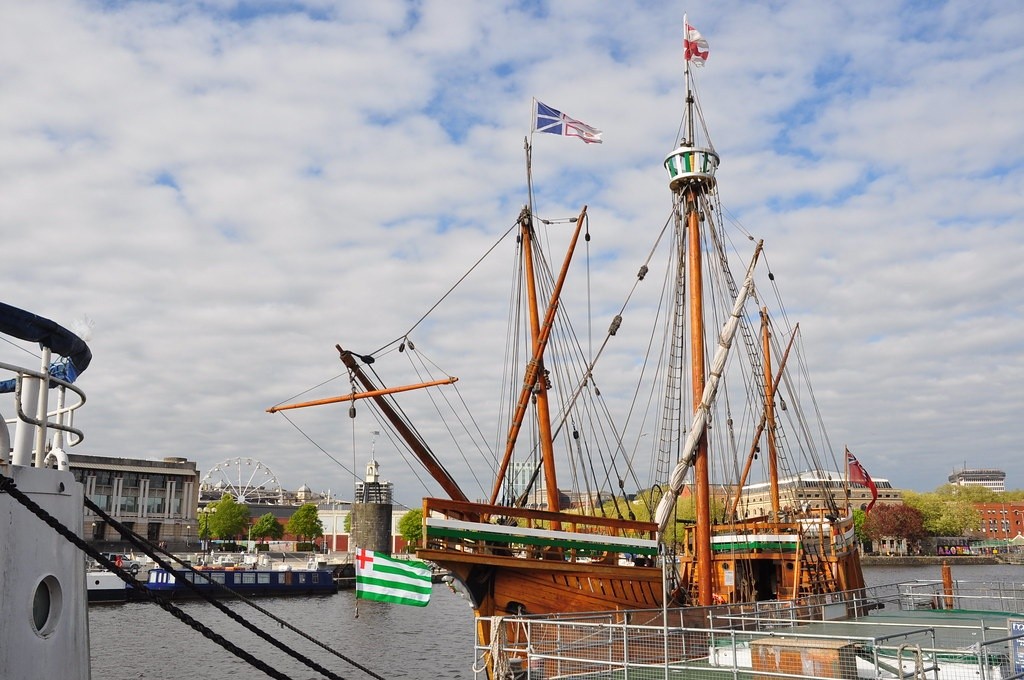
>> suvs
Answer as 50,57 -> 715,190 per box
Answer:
93,552 -> 143,575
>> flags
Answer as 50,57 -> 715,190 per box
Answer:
354,543 -> 432,608
534,99 -> 603,144
846,447 -> 878,518
684,18 -> 711,67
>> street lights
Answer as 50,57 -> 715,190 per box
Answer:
198,507 -> 217,566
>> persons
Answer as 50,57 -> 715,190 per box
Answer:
282,552 -> 286,562
115,555 -> 123,567
158,540 -> 167,550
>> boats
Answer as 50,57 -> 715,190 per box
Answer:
265,14 -> 1024,679
124,562 -> 339,600
